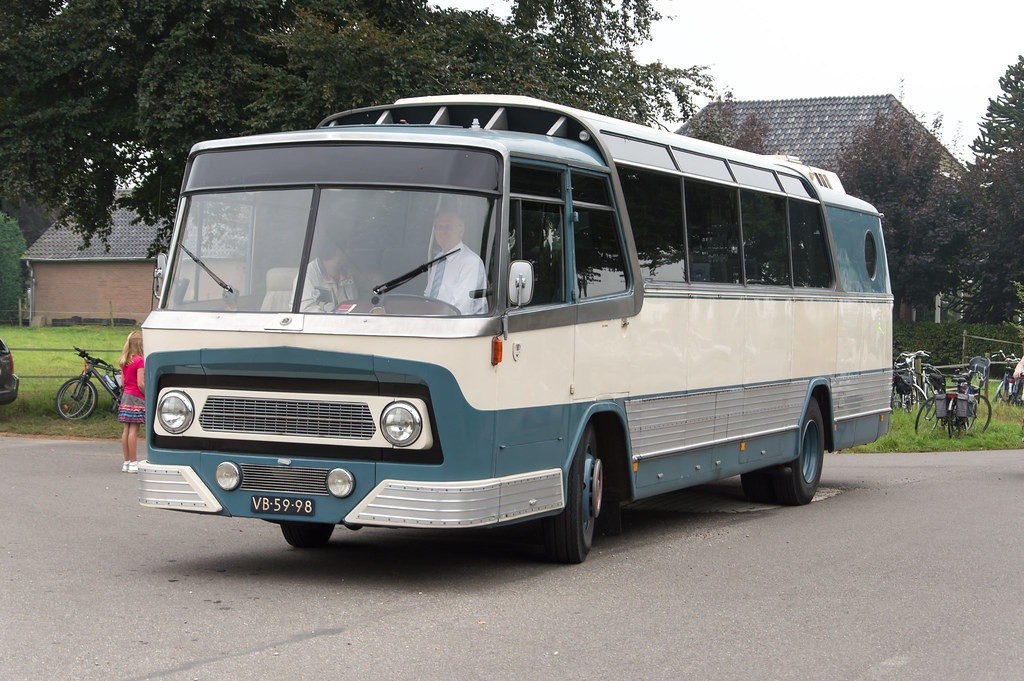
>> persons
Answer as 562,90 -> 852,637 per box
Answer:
420,210 -> 488,315
117,330 -> 144,475
1013,355 -> 1024,401
290,224 -> 358,314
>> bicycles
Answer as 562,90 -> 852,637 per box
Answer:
56,346 -> 123,420
890,350 -> 1024,438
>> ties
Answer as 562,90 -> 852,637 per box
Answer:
427,251 -> 450,303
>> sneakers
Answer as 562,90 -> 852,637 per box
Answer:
122,458 -> 148,474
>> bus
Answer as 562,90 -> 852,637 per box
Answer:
139,93 -> 894,566
